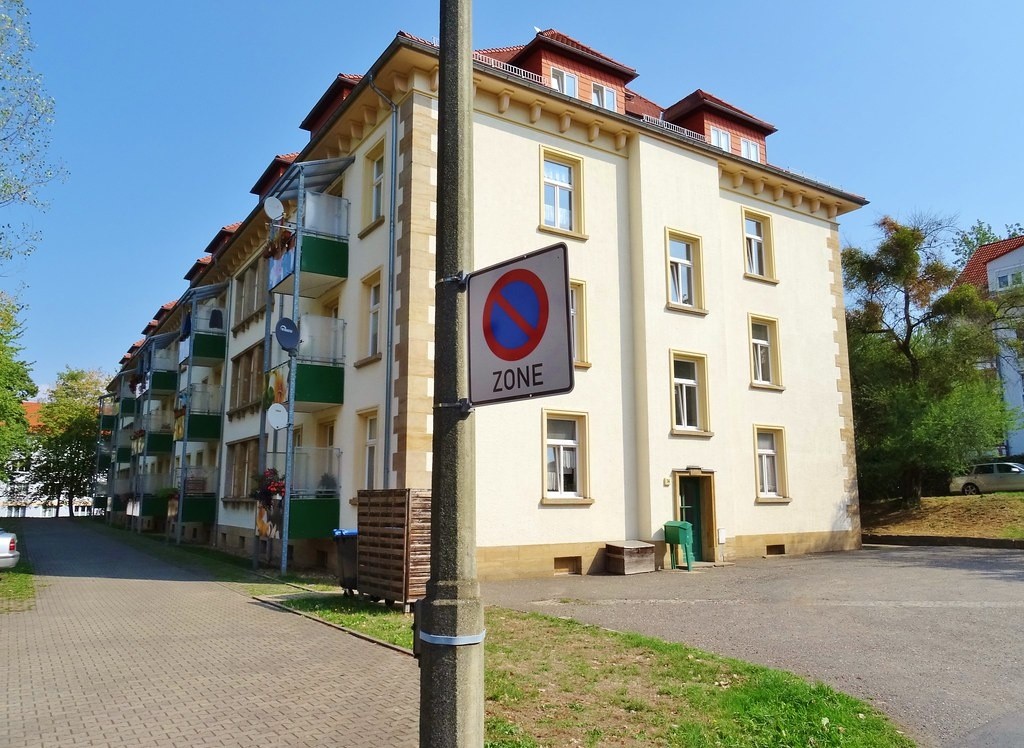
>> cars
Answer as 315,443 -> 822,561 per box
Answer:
960,460 -> 1024,496
1,529 -> 20,572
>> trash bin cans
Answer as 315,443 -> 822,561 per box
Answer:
336,529 -> 358,597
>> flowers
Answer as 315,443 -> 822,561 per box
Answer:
250,467 -> 285,512
133,429 -> 145,435
174,404 -> 186,418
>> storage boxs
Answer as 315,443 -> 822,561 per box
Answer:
605,540 -> 655,575
